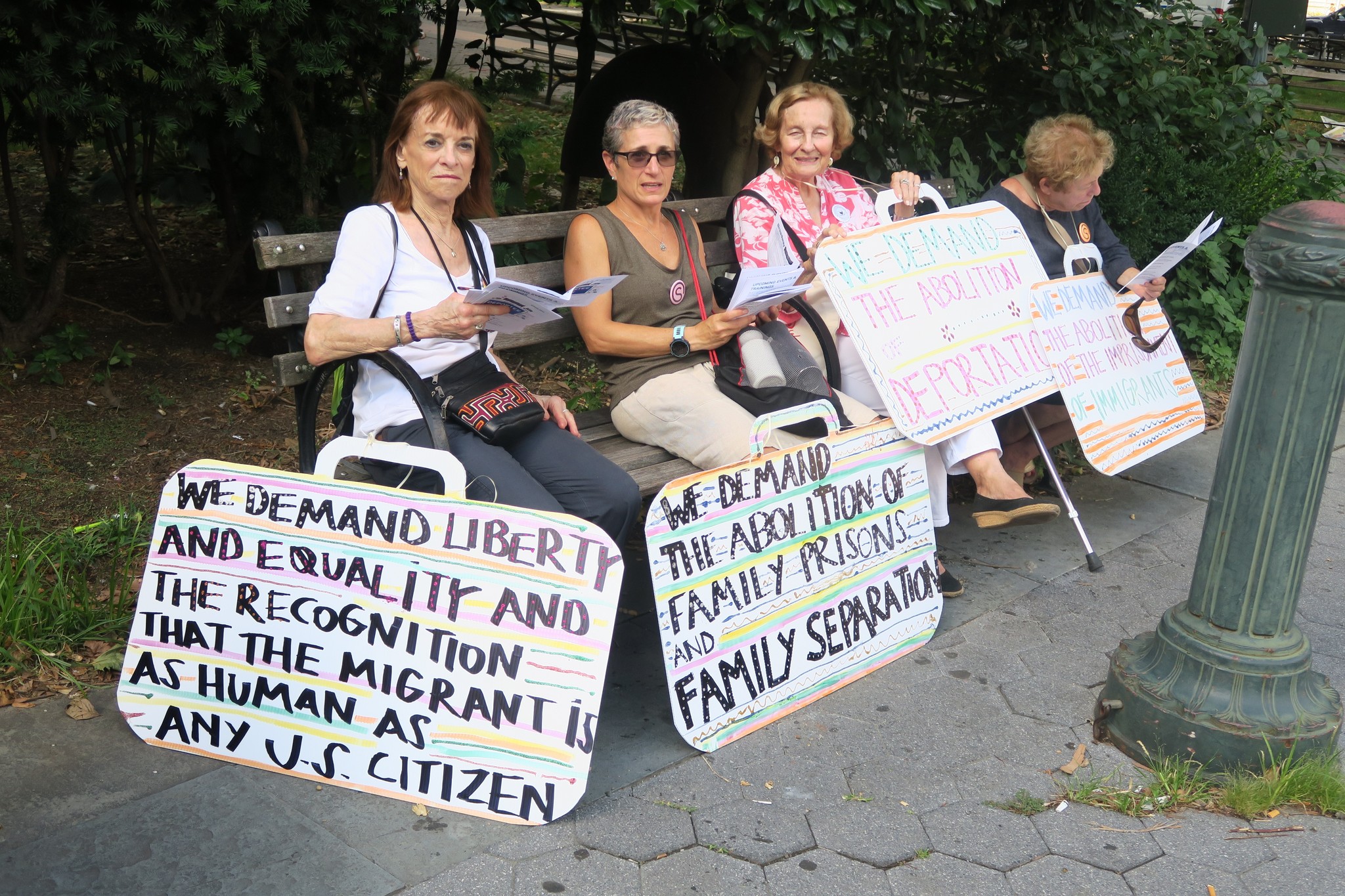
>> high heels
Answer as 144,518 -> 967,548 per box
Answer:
939,568 -> 964,597
971,493 -> 1060,529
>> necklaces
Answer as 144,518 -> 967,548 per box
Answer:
421,219 -> 460,257
614,198 -> 667,251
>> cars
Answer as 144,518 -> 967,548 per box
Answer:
1301,6 -> 1345,55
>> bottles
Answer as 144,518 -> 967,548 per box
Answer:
762,320 -> 823,392
738,325 -> 787,389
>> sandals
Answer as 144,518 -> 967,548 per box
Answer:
1022,459 -> 1037,484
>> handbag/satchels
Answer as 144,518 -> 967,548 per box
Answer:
419,350 -> 546,448
711,189 -> 841,379
708,329 -> 855,438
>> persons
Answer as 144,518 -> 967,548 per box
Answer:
564,100 -> 882,470
303,78 -> 643,559
735,82 -> 1061,597
404,0 -> 432,64
976,113 -> 1165,490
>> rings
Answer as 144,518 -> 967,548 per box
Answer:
475,323 -> 483,331
563,409 -> 569,412
900,179 -> 909,187
816,242 -> 821,248
913,184 -> 920,189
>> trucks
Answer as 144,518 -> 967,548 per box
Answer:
1133,0 -> 1239,35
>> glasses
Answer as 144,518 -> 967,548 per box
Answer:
1122,280 -> 1172,353
610,150 -> 683,168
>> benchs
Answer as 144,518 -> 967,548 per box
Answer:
490,8 -> 714,105
255,180 -> 957,498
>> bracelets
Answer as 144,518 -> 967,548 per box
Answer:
405,312 -> 421,341
394,315 -> 405,347
893,209 -> 917,220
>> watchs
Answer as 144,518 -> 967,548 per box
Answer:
670,324 -> 690,360
